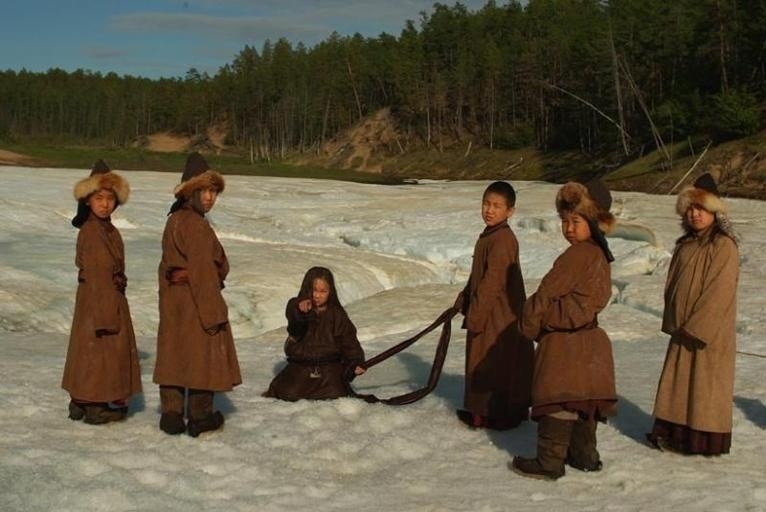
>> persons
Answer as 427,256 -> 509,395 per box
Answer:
453,182 -> 535,431
513,182 -> 618,478
646,172 -> 739,455
61,162 -> 143,425
154,153 -> 242,436
263,267 -> 371,400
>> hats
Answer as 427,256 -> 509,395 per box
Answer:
74,159 -> 130,205
675,174 -> 728,217
555,175 -> 613,227
174,152 -> 225,197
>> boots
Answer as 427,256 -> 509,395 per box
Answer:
160,386 -> 186,434
69,401 -> 84,421
566,418 -> 603,473
513,415 -> 576,480
84,403 -> 128,424
187,388 -> 225,437
